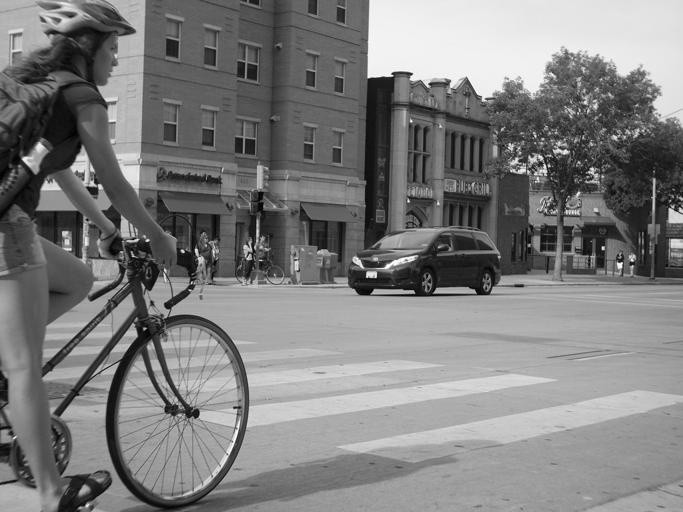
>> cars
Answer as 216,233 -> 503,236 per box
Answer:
348,226 -> 502,295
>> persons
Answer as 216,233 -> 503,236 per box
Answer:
242,236 -> 255,285
0,1 -> 178,512
628,251 -> 636,276
249,235 -> 271,283
616,250 -> 624,277
194,232 -> 216,285
207,236 -> 221,280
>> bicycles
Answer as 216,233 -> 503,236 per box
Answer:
235,250 -> 285,285
0,236 -> 249,512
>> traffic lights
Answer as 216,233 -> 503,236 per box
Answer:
250,190 -> 263,214
263,167 -> 269,187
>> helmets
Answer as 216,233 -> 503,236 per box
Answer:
35,0 -> 136,36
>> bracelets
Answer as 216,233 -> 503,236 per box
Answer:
98,226 -> 117,242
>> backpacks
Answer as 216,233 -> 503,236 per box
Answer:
0,57 -> 99,220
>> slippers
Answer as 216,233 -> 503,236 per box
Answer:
40,469 -> 113,512
